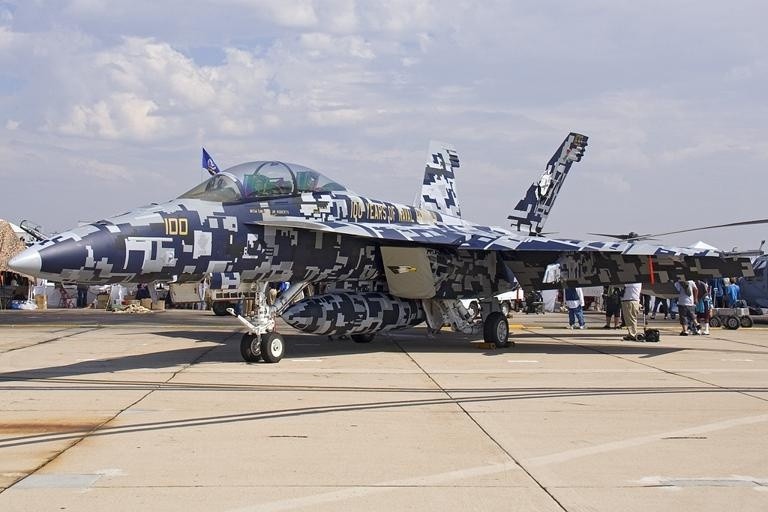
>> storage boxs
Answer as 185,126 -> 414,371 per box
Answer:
128,298 -> 166,310
36,294 -> 48,310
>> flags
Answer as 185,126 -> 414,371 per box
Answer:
202,147 -> 220,176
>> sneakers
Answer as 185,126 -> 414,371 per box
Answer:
623,334 -> 636,340
566,325 -> 573,329
680,322 -> 710,335
580,325 -> 588,329
603,322 -> 625,329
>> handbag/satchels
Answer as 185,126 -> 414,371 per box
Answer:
602,294 -> 619,305
749,307 -> 763,315
731,300 -> 747,308
645,328 -> 659,342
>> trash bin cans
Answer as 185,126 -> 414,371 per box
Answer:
32,284 -> 49,311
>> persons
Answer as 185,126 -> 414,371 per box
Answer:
526,276 -> 742,341
136,283 -> 148,299
76,283 -> 90,308
